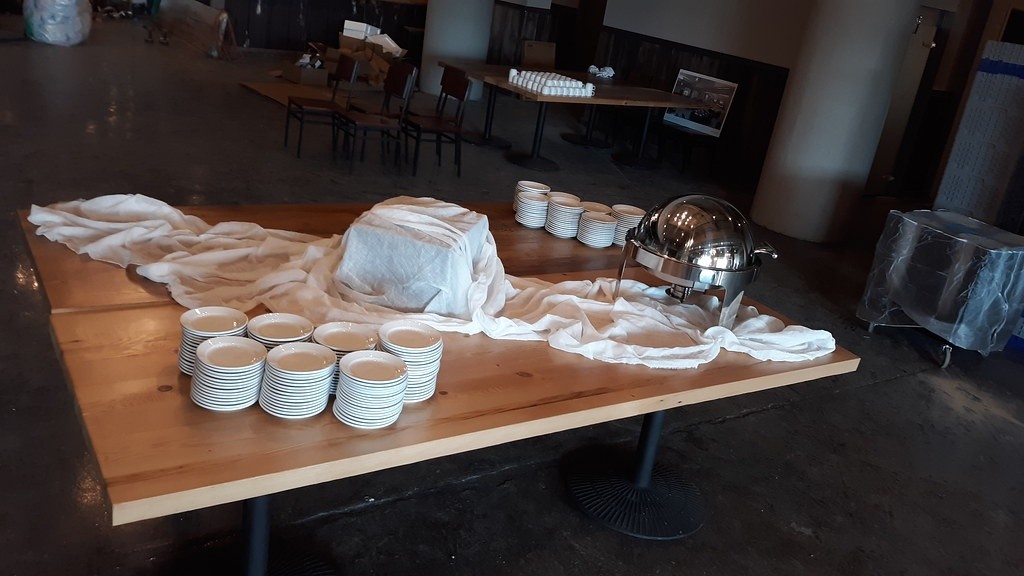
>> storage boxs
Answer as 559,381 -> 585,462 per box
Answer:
520,39 -> 557,68
282,17 -> 409,88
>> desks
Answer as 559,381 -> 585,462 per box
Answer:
437,61 -> 703,173
16,193 -> 863,576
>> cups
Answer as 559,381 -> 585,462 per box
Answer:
508,69 -> 596,97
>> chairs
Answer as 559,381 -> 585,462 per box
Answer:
334,63 -> 411,177
280,54 -> 360,159
346,58 -> 409,160
402,61 -> 458,154
400,75 -> 473,179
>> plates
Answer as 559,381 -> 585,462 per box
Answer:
176,306 -> 444,429
513,181 -> 647,248
